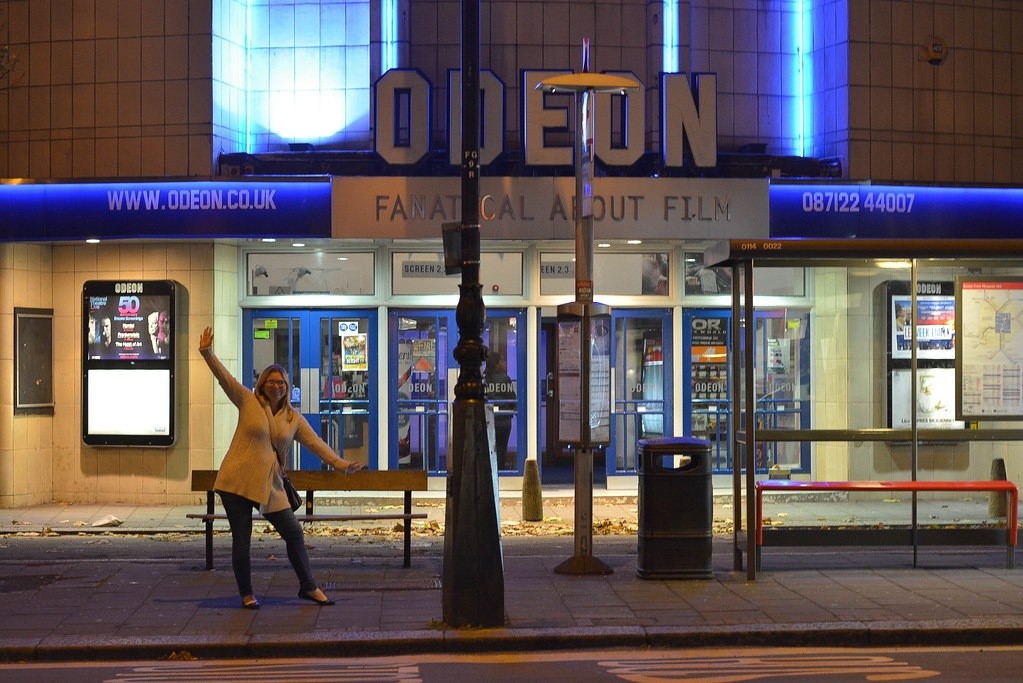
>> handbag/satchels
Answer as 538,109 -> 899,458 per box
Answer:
283,476 -> 303,511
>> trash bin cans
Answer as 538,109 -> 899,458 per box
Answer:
635,437 -> 717,580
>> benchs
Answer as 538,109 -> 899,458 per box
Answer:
756,480 -> 1019,570
186,469 -> 428,567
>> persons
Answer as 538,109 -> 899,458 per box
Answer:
484,352 -> 516,470
95,316 -> 111,344
141,311 -> 170,356
199,326 -> 364,609
896,304 -> 907,331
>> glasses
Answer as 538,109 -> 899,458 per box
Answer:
264,379 -> 286,386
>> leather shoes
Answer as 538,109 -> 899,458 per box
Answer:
240,591 -> 259,608
298,589 -> 335,606
